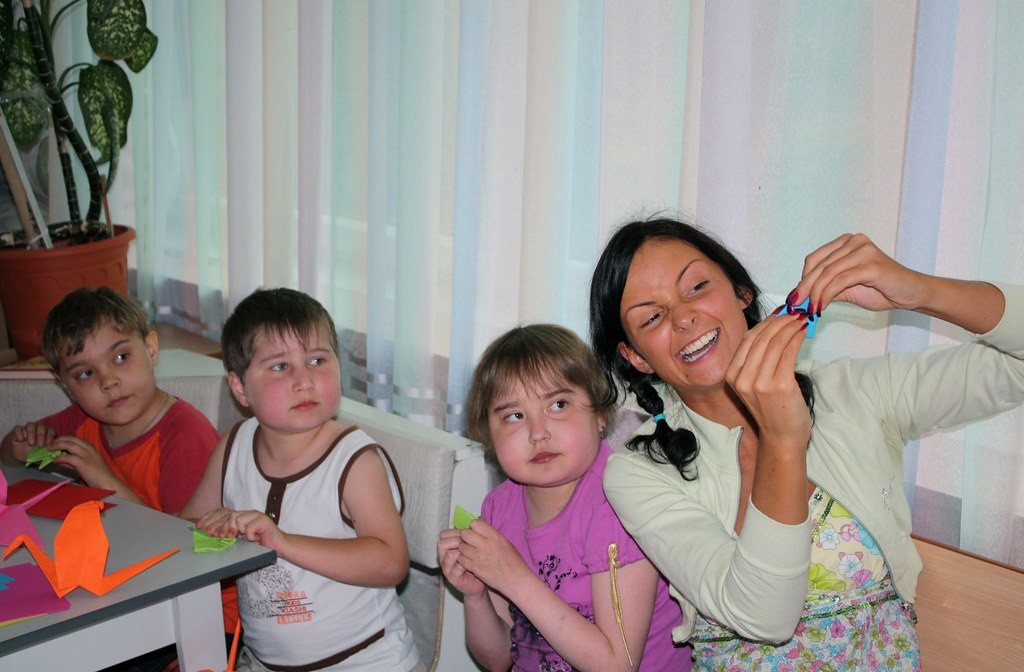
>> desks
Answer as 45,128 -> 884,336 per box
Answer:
0,458 -> 276,672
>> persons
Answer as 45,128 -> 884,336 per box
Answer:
439,324 -> 696,672
589,217 -> 1024,672
177,286 -> 426,672
0,286 -> 244,672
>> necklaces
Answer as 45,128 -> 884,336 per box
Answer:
105,390 -> 169,449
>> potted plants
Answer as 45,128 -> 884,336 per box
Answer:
0,0 -> 158,359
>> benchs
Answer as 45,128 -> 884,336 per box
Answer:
910,534 -> 1024,672
0,374 -> 453,672
